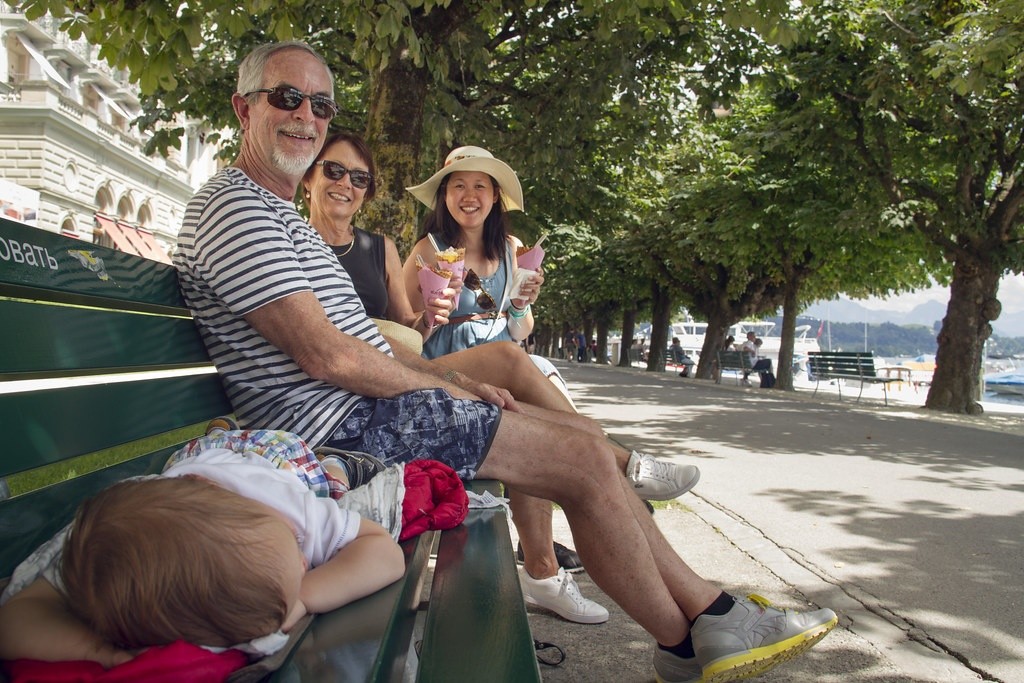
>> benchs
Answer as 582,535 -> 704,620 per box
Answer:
713,351 -> 767,387
665,349 -> 690,378
628,349 -> 647,368
1,221 -> 543,683
805,352 -> 903,407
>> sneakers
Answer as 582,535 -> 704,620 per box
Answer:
623,452 -> 699,501
517,537 -> 585,572
652,642 -> 705,682
691,593 -> 838,683
520,565 -> 609,623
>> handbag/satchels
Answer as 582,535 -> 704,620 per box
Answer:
760,372 -> 777,388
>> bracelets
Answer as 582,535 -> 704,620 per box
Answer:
508,299 -> 530,319
422,311 -> 439,329
444,370 -> 458,382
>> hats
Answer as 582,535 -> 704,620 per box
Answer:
405,145 -> 525,212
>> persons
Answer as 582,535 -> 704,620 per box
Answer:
400,145 -> 656,574
563,328 -> 597,362
294,131 -> 700,623
631,337 -> 648,365
668,337 -> 694,377
174,43 -> 837,683
525,332 -> 536,354
723,330 -> 774,386
0,417 -> 408,666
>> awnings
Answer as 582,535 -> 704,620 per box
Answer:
138,231 -> 173,265
118,100 -> 155,139
96,216 -> 140,255
16,32 -> 71,90
118,223 -> 158,260
92,83 -> 131,123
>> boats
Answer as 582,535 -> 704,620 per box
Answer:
984,372 -> 1024,395
638,320 -> 825,376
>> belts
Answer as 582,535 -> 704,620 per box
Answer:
448,311 -> 507,322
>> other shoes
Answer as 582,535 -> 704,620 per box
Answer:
679,373 -> 688,377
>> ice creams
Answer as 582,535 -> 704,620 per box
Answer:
416,261 -> 452,279
434,246 -> 465,263
516,245 -> 534,257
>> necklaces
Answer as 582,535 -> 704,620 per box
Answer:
336,225 -> 355,257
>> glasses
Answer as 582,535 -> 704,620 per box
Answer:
243,86 -> 337,118
315,159 -> 374,189
465,269 -> 498,310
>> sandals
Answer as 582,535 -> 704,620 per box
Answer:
313,446 -> 386,490
206,416 -> 238,436
